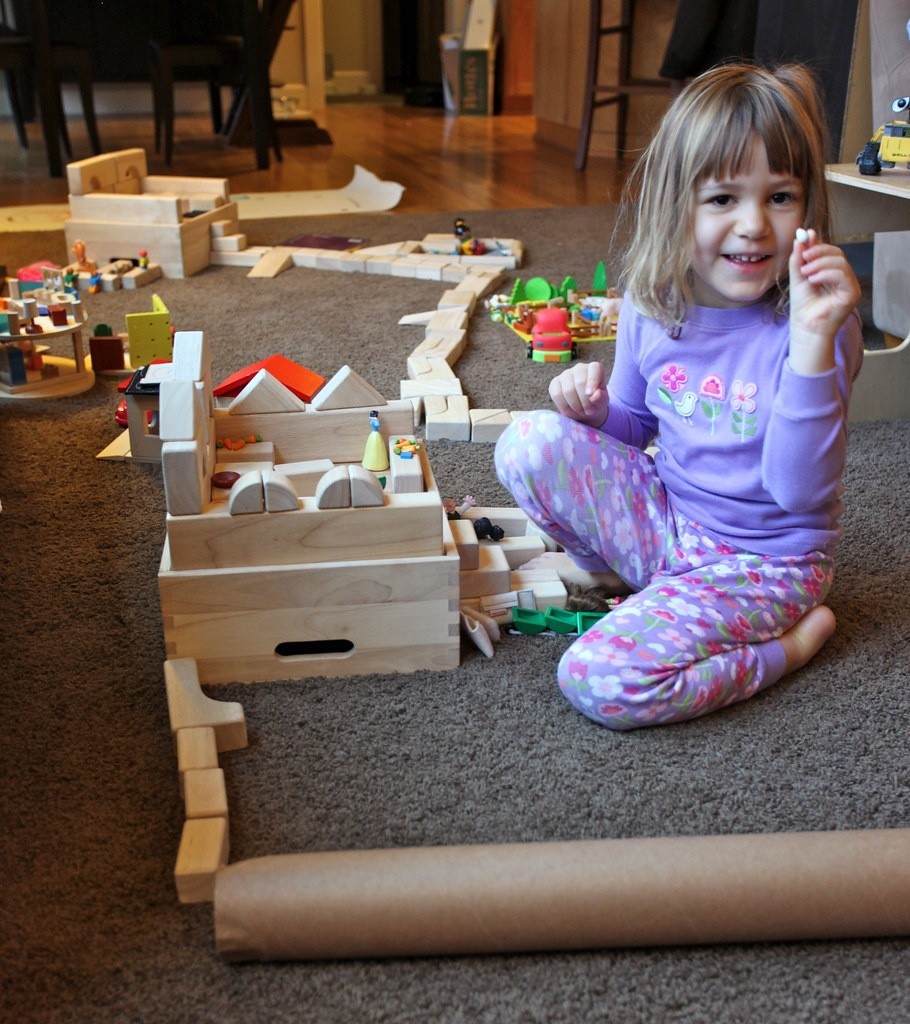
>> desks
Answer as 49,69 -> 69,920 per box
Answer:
823,162 -> 910,424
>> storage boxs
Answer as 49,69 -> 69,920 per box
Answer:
157,506 -> 460,687
461,0 -> 497,50
166,438 -> 442,571
441,32 -> 499,115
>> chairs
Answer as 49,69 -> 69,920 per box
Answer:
152,0 -> 292,171
0,0 -> 104,177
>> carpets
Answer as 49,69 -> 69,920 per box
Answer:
0,204 -> 910,1024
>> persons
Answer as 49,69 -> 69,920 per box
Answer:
494,62 -> 866,733
